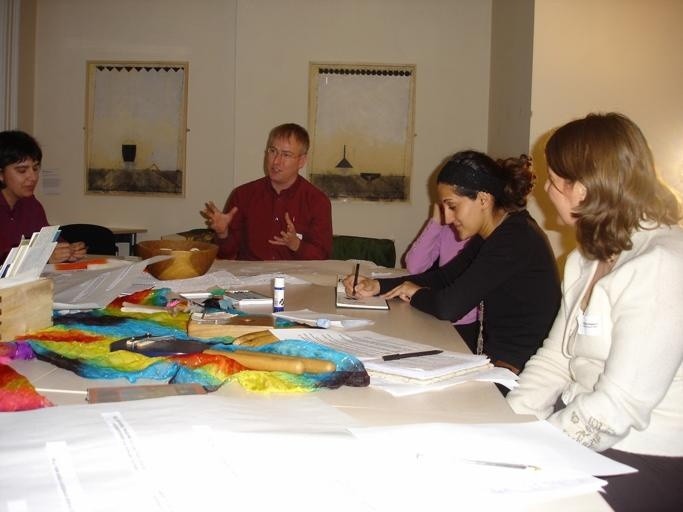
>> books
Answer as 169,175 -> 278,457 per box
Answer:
335,273 -> 389,309
0,225 -> 63,284
356,348 -> 490,380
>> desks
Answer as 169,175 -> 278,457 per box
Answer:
1,257 -> 615,512
105,226 -> 148,257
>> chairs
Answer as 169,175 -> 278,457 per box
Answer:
56,223 -> 118,256
333,236 -> 396,268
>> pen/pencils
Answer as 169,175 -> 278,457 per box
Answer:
353,264 -> 360,296
382,350 -> 444,361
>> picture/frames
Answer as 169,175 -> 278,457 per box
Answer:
86,58 -> 188,198
307,60 -> 417,203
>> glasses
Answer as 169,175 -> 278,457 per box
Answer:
268,147 -> 306,158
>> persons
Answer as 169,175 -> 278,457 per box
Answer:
343,151 -> 561,396
405,201 -> 480,355
504,112 -> 683,511
204,123 -> 333,261
0,131 -> 87,268
